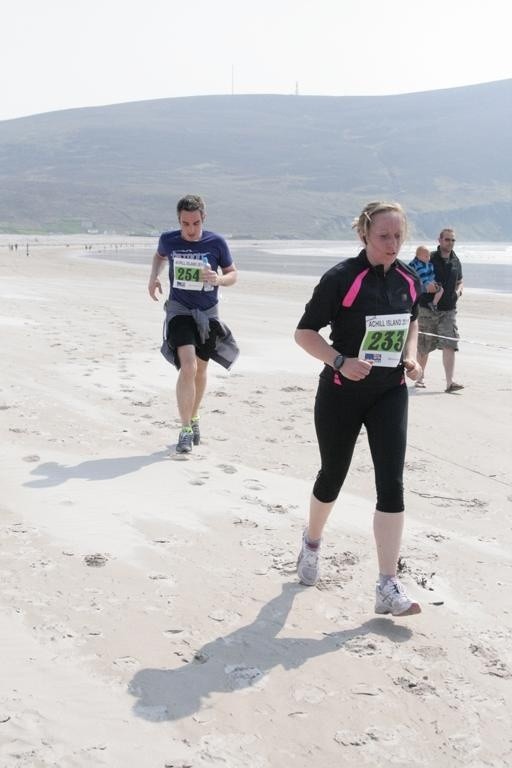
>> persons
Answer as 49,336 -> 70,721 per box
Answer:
150,195 -> 238,452
416,229 -> 463,392
409,246 -> 444,315
294,201 -> 424,617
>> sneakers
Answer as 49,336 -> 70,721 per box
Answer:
296,527 -> 321,586
375,577 -> 421,616
176,416 -> 200,453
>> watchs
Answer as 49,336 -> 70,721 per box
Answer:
332,353 -> 346,374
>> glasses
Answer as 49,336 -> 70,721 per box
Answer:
445,237 -> 456,242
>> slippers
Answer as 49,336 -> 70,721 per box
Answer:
445,383 -> 463,392
415,382 -> 426,388
